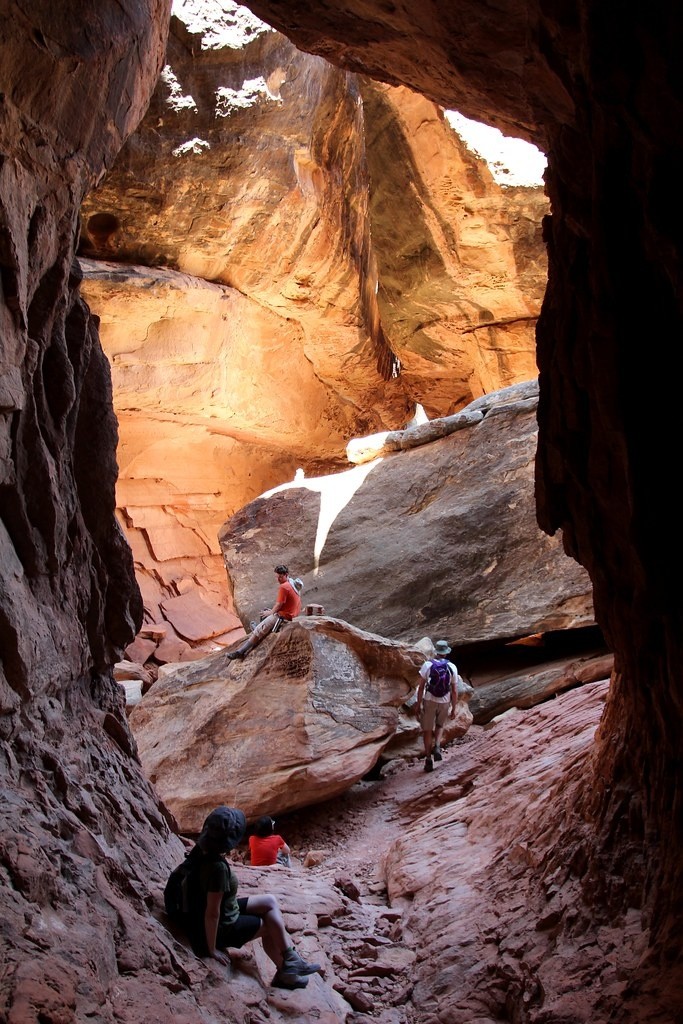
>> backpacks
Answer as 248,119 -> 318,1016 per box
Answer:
164,845 -> 200,936
428,659 -> 450,697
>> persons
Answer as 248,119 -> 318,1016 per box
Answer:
248,816 -> 293,870
226,564 -> 302,662
414,641 -> 458,772
164,806 -> 321,991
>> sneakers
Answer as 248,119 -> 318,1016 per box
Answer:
226,651 -> 244,659
423,759 -> 433,771
282,956 -> 320,975
249,621 -> 257,631
271,973 -> 308,989
434,746 -> 442,762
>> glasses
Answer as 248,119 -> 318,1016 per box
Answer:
272,821 -> 275,824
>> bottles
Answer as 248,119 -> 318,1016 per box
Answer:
272,616 -> 284,633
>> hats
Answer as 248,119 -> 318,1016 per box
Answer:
197,806 -> 245,852
434,640 -> 451,654
288,577 -> 303,596
